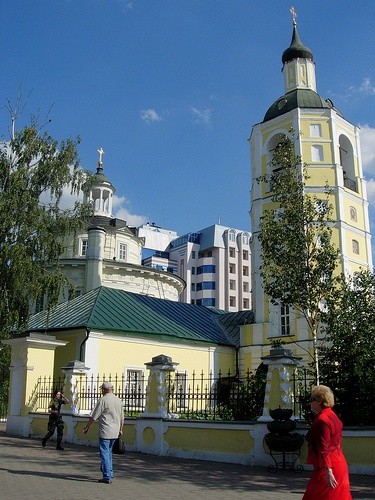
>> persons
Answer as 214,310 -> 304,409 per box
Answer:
301,385 -> 353,500
42,390 -> 69,450
83,382 -> 125,483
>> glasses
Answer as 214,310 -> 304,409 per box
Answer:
310,397 -> 316,403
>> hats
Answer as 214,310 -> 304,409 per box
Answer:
100,382 -> 112,389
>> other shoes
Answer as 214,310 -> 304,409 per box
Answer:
56,446 -> 64,450
98,479 -> 112,484
42,438 -> 47,447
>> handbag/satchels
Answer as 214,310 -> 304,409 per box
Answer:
112,433 -> 125,455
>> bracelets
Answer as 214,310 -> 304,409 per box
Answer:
63,396 -> 66,398
